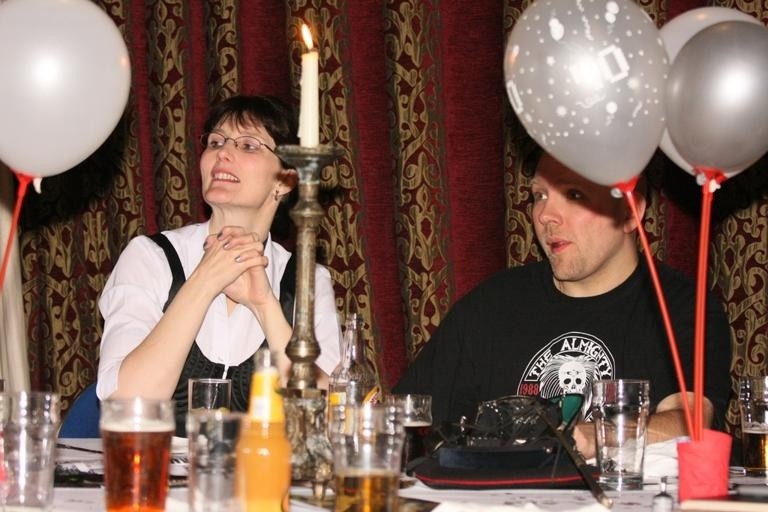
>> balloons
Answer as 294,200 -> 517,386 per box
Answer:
669,19 -> 767,183
657,6 -> 767,186
503,1 -> 670,196
0,0 -> 132,190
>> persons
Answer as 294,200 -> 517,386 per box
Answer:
96,95 -> 342,445
391,146 -> 732,466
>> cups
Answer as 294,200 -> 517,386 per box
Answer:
331,391 -> 437,511
589,377 -> 652,485
737,375 -> 768,469
1,377 -> 234,512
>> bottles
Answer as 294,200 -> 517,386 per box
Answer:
328,311 -> 381,440
232,348 -> 293,511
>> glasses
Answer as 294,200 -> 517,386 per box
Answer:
199,132 -> 282,162
